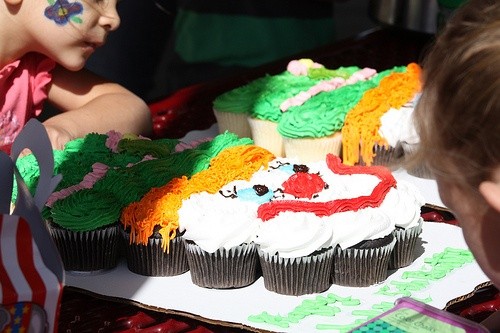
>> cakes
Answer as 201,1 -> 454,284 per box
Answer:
10,130 -> 425,296
212,59 -> 437,180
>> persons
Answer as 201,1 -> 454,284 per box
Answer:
0,0 -> 152,164
410,1 -> 500,291
